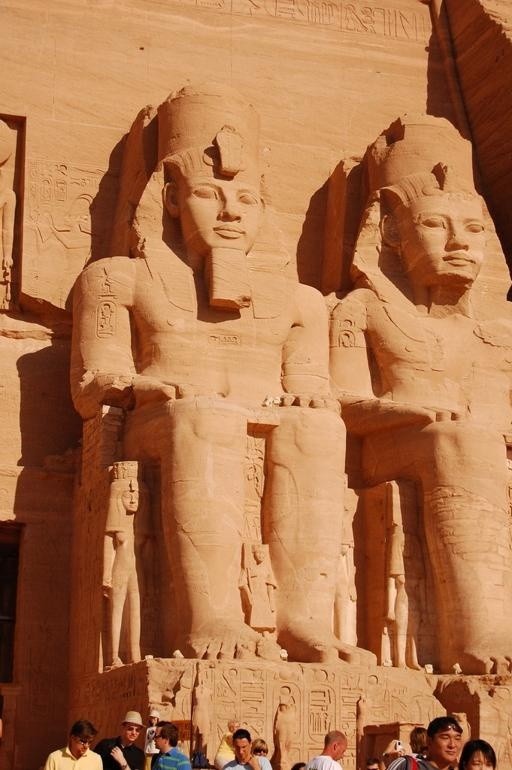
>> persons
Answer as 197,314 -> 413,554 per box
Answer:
241,540 -> 278,639
68,85 -> 381,668
103,458 -> 156,669
386,477 -> 425,670
326,113 -> 511,673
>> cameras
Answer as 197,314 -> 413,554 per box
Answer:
396,741 -> 402,751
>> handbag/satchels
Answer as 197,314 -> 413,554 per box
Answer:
192,751 -> 209,768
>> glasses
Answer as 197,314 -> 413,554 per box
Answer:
435,724 -> 463,734
254,748 -> 267,754
153,733 -> 162,739
124,724 -> 142,733
79,736 -> 93,745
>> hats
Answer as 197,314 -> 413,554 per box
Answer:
148,710 -> 160,719
120,711 -> 146,727
